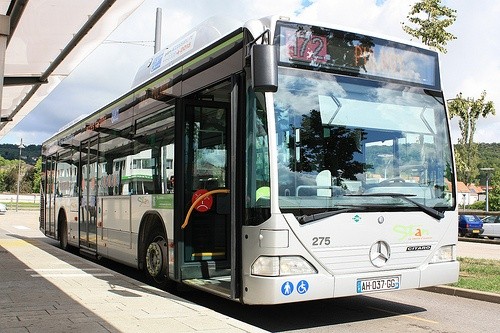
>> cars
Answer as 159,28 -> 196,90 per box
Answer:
459,214 -> 485,237
478,214 -> 500,239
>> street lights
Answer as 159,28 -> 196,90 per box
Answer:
16,137 -> 25,212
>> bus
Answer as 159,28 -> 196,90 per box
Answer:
39,13 -> 461,305
